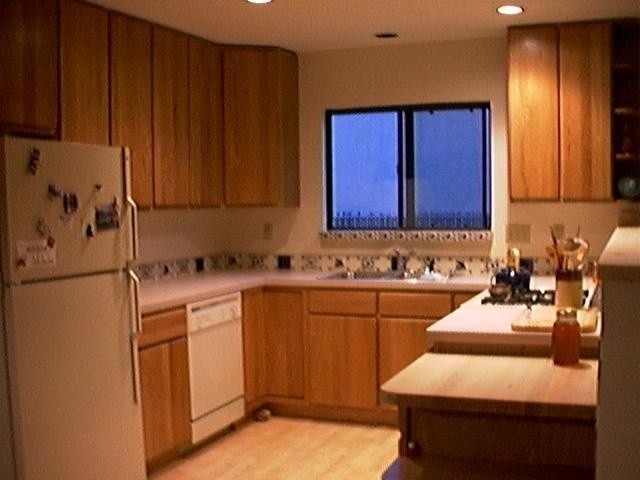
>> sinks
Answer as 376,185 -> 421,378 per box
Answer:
324,270 -> 386,282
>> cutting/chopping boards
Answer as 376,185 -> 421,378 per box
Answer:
511,304 -> 598,334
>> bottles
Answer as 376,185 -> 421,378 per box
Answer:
550,307 -> 581,366
514,266 -> 530,293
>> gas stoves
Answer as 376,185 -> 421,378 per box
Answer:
480,288 -> 589,307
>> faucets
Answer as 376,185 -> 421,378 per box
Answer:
393,249 -> 404,278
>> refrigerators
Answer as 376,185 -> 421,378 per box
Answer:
1,135 -> 142,477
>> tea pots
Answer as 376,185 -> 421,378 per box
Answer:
488,270 -> 512,299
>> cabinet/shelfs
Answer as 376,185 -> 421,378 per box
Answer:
60,3 -> 301,208
0,0 -> 58,138
243,285 -> 482,429
137,307 -> 193,476
610,16 -> 640,199
508,19 -> 614,201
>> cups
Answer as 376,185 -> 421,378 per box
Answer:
554,270 -> 584,314
